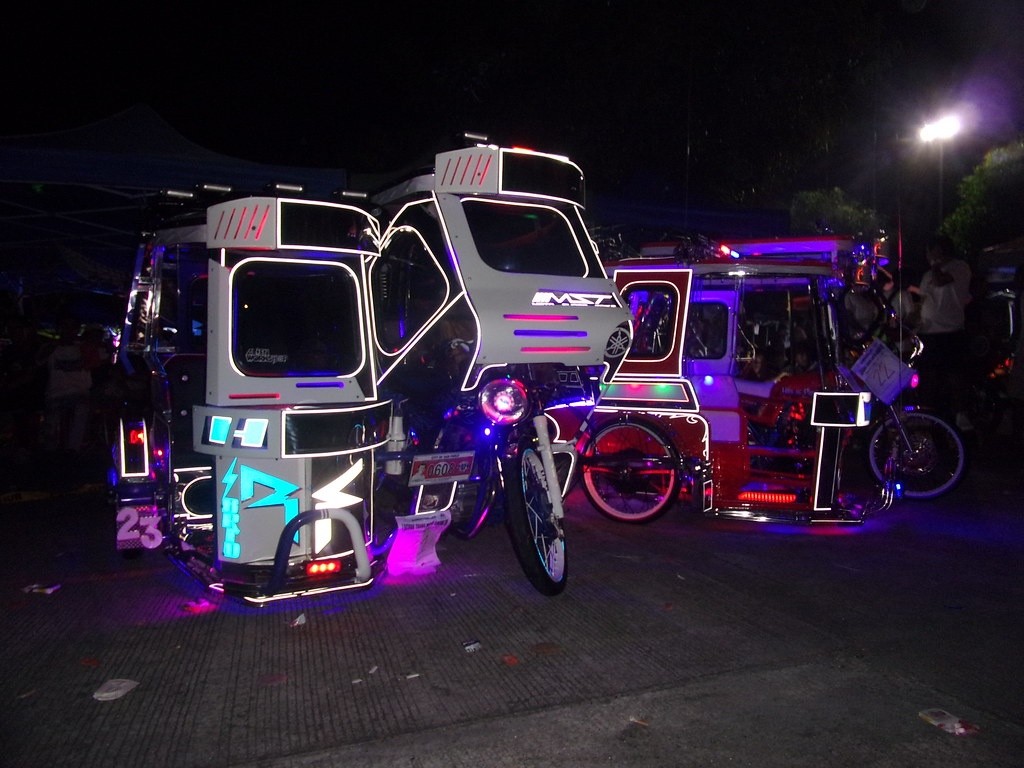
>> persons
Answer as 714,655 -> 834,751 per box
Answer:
634,234 -> 974,421
298,338 -> 329,369
0,314 -> 134,476
160,268 -> 186,319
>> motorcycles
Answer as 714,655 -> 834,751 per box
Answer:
88,142 -> 635,611
569,235 -> 1016,531
